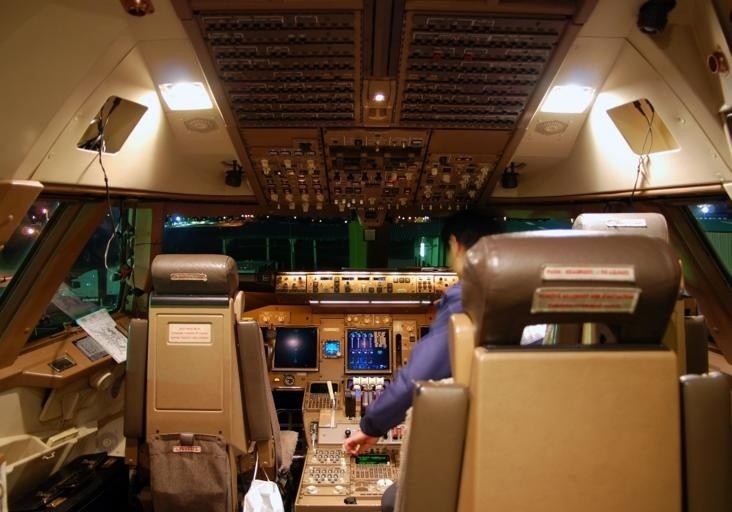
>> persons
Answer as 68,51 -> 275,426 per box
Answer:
339,208 -> 552,463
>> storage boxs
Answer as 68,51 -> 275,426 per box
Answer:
14,453 -> 125,512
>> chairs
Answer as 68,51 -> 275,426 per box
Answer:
121,252 -> 299,511
391,228 -> 731,512
